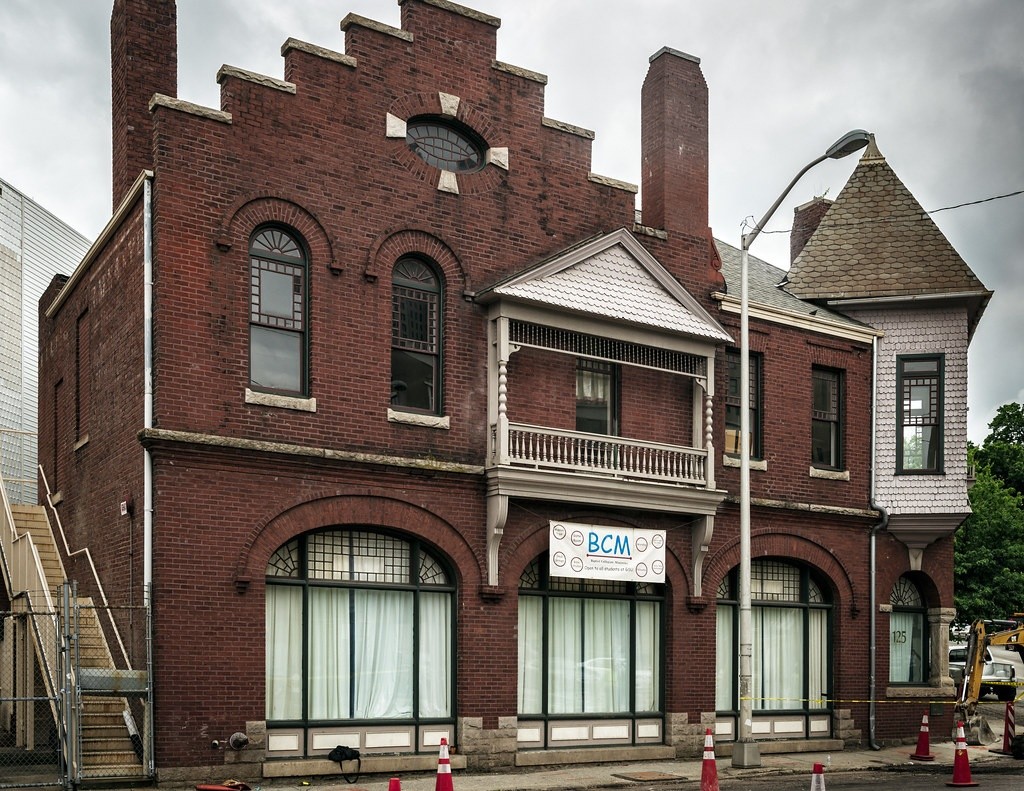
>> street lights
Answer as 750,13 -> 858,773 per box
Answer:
732,128 -> 871,769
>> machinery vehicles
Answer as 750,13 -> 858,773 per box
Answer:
950,611 -> 1024,759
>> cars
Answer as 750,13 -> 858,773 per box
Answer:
950,622 -> 971,642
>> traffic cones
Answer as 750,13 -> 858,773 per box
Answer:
810,762 -> 827,791
908,708 -> 936,762
989,703 -> 1017,756
945,721 -> 981,786
434,737 -> 454,791
699,728 -> 720,791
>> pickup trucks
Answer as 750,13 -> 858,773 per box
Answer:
948,645 -> 1017,701
